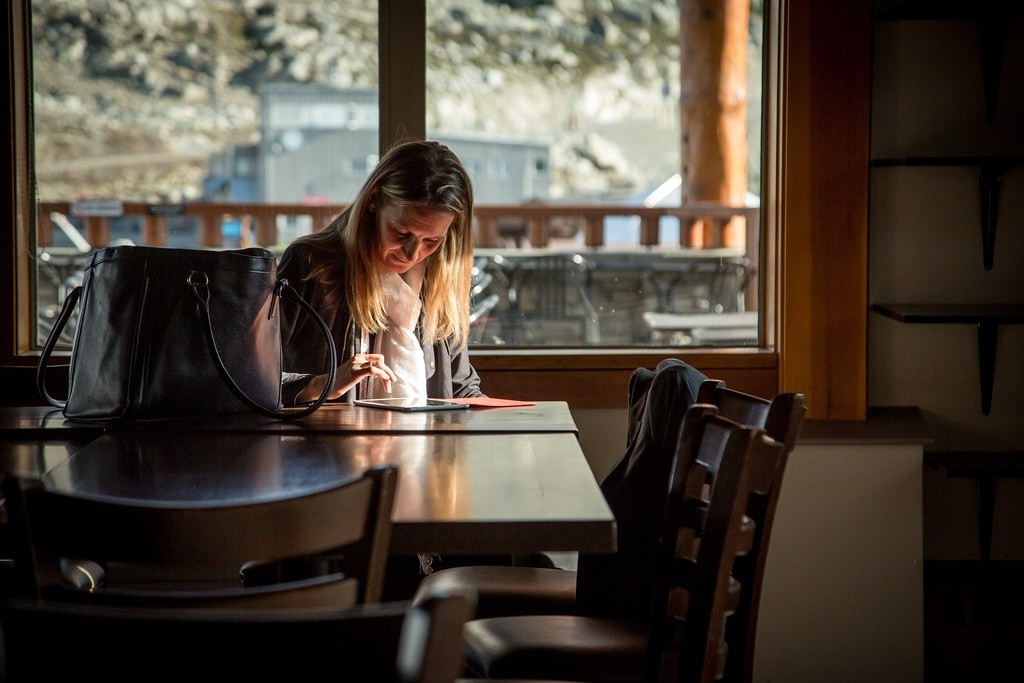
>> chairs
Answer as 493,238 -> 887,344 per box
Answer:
0,463 -> 402,609
471,251 -> 757,348
460,403 -> 787,682
0,601 -> 415,683
395,357 -> 708,682
693,379 -> 808,683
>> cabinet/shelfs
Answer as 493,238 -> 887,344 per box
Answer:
867,0 -> 1024,563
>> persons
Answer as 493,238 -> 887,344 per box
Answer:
274,139 -> 490,404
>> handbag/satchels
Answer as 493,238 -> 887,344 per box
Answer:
37,246 -> 338,430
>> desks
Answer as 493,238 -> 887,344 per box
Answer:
101,400 -> 580,444
1,407 -> 109,496
0,432 -> 619,602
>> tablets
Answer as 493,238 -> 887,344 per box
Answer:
358,395 -> 470,412
